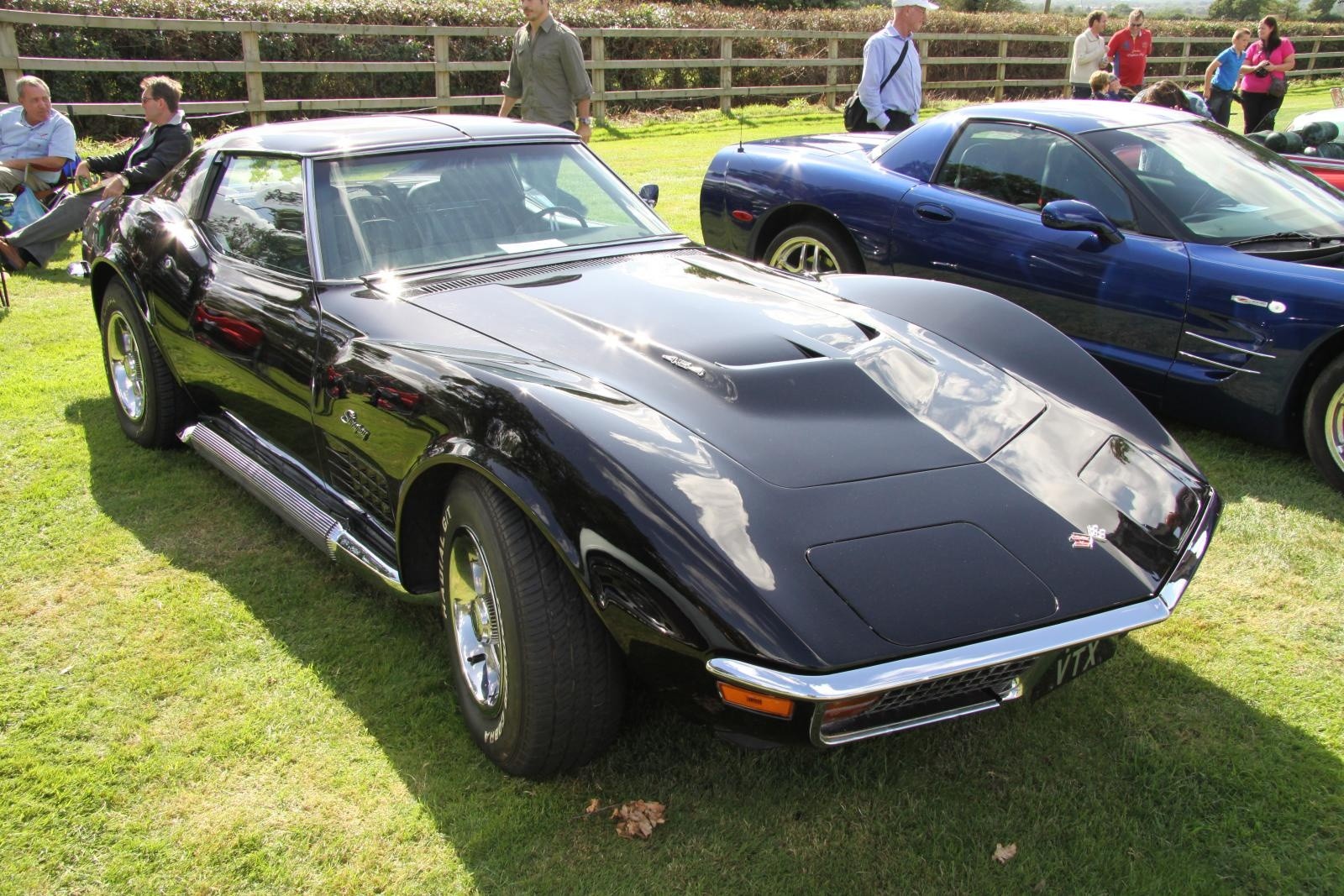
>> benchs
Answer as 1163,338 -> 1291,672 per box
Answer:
256,180 -> 419,275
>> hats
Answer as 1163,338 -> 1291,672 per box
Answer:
892,0 -> 939,11
1108,73 -> 1116,82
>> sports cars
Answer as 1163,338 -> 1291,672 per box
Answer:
696,94 -> 1344,498
68,111 -> 1230,785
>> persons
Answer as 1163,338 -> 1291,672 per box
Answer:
1069,11 -> 1110,100
858,0 -> 939,132
1107,9 -> 1152,94
0,75 -> 77,197
0,76 -> 194,271
317,365 -> 343,417
1131,79 -> 1215,176
1239,16 -> 1295,137
498,0 -> 595,205
1203,28 -> 1250,127
1088,70 -> 1136,102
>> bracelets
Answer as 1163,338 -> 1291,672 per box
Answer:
578,117 -> 590,124
117,175 -> 129,187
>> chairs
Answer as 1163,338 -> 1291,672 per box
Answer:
1302,121 -> 1344,159
11,158 -> 75,212
333,196 -> 415,265
955,143 -> 1013,204
1264,132 -> 1306,155
1039,140 -> 1101,212
407,168 -> 519,246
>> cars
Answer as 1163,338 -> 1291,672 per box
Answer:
1111,106 -> 1344,197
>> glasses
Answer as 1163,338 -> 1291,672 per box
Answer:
1129,22 -> 1142,27
140,97 -> 160,104
906,6 -> 926,14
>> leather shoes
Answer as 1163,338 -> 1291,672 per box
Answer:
0,237 -> 26,271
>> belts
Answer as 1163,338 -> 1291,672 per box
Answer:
1211,86 -> 1233,95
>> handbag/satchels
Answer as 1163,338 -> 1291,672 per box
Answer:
1270,80 -> 1284,99
0,187 -> 45,231
844,89 -> 867,132
